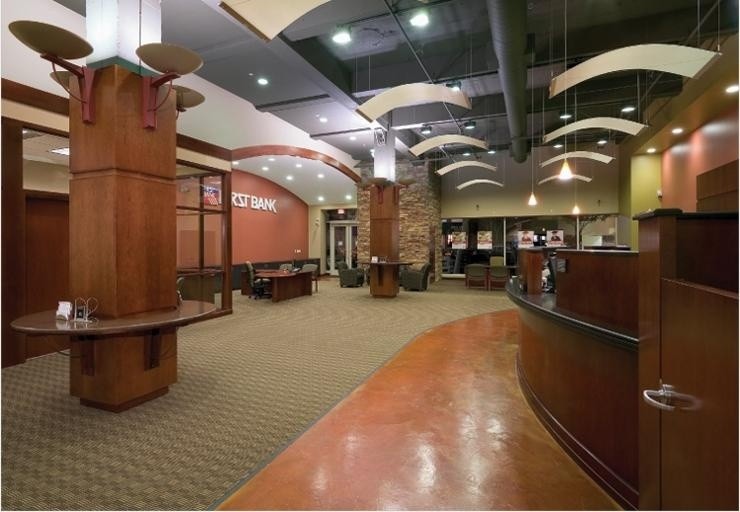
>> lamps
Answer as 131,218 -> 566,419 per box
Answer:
420,78 -> 479,136
528,46 -> 537,208
327,17 -> 355,49
559,1 -> 576,189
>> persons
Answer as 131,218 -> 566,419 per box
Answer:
522,231 -> 531,241
551,231 -> 561,241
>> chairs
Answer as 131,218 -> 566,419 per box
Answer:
242,261 -> 322,303
466,254 -> 512,292
334,260 -> 432,300
